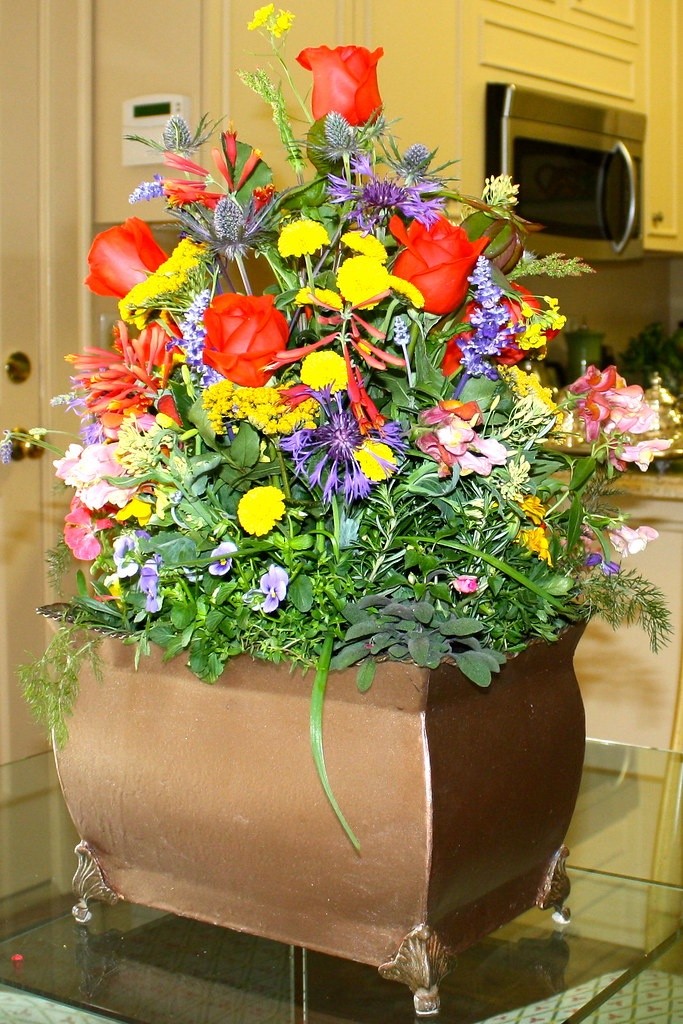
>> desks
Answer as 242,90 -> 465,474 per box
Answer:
0,736 -> 683,1024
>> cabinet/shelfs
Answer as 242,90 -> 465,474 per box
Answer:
637,0 -> 683,254
333,0 -> 487,228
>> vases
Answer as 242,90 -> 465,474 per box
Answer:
35,602 -> 603,1020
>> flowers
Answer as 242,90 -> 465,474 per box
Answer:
0,3 -> 674,851
